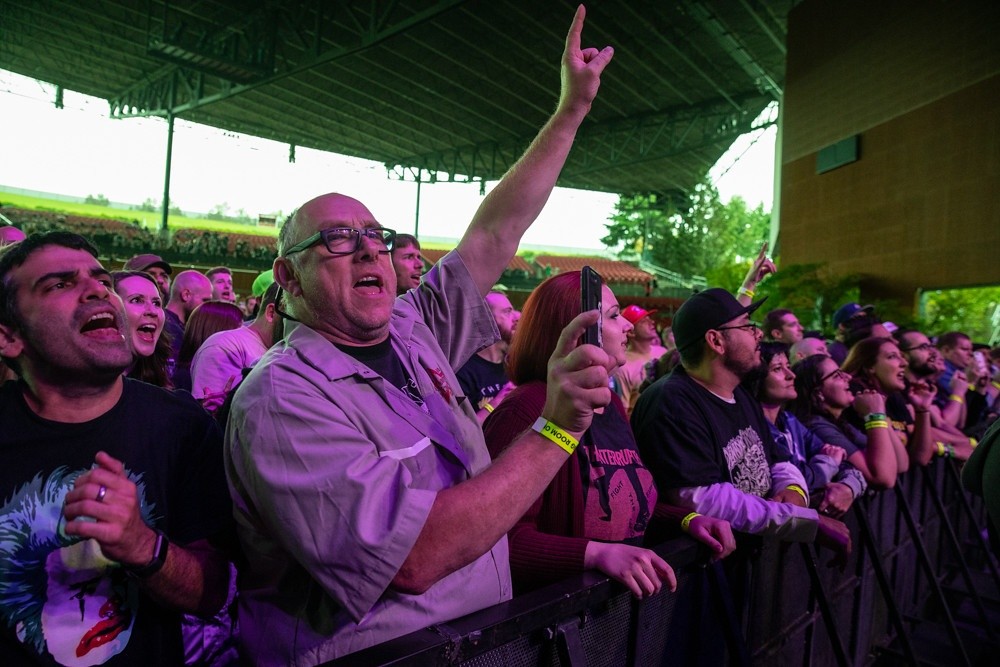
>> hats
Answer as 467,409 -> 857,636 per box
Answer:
805,330 -> 827,340
835,303 -> 875,324
620,306 -> 658,325
122,254 -> 173,276
671,288 -> 769,352
252,269 -> 275,298
881,321 -> 898,333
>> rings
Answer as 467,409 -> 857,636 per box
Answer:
96,484 -> 105,502
825,508 -> 831,515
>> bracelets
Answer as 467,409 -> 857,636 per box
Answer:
935,441 -> 945,456
484,405 -> 493,413
947,394 -> 964,404
737,285 -> 754,298
969,437 -> 977,448
864,411 -> 886,423
680,513 -> 703,530
146,529 -> 168,576
787,486 -> 808,509
865,421 -> 887,428
946,444 -> 954,457
532,417 -> 578,454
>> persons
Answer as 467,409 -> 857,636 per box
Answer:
0,214 -> 1000,599
0,232 -> 245,667
223,4 -> 613,667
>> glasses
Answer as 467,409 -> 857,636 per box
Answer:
906,343 -> 938,353
714,323 -> 759,339
817,369 -> 844,383
283,227 -> 397,257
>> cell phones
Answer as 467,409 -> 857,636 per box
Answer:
580,266 -> 602,366
